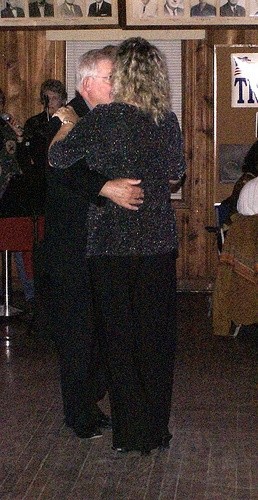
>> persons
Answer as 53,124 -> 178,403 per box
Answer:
0,36 -> 185,452
190,0 -> 217,17
133,0 -> 159,19
220,0 -> 247,16
164,0 -> 184,17
1,0 -> 25,18
57,0 -> 83,17
29,0 -> 54,17
87,0 -> 112,17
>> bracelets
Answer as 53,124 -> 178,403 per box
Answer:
16,134 -> 24,137
61,121 -> 75,125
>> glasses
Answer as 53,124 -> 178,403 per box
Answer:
91,75 -> 113,81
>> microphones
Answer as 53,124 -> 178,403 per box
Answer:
2,113 -> 21,130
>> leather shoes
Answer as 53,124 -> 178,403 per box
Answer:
87,404 -> 112,429
65,409 -> 102,439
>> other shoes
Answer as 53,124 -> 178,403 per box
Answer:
229,322 -> 241,337
0,302 -> 25,319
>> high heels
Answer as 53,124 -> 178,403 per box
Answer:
153,433 -> 173,450
111,439 -> 158,457
